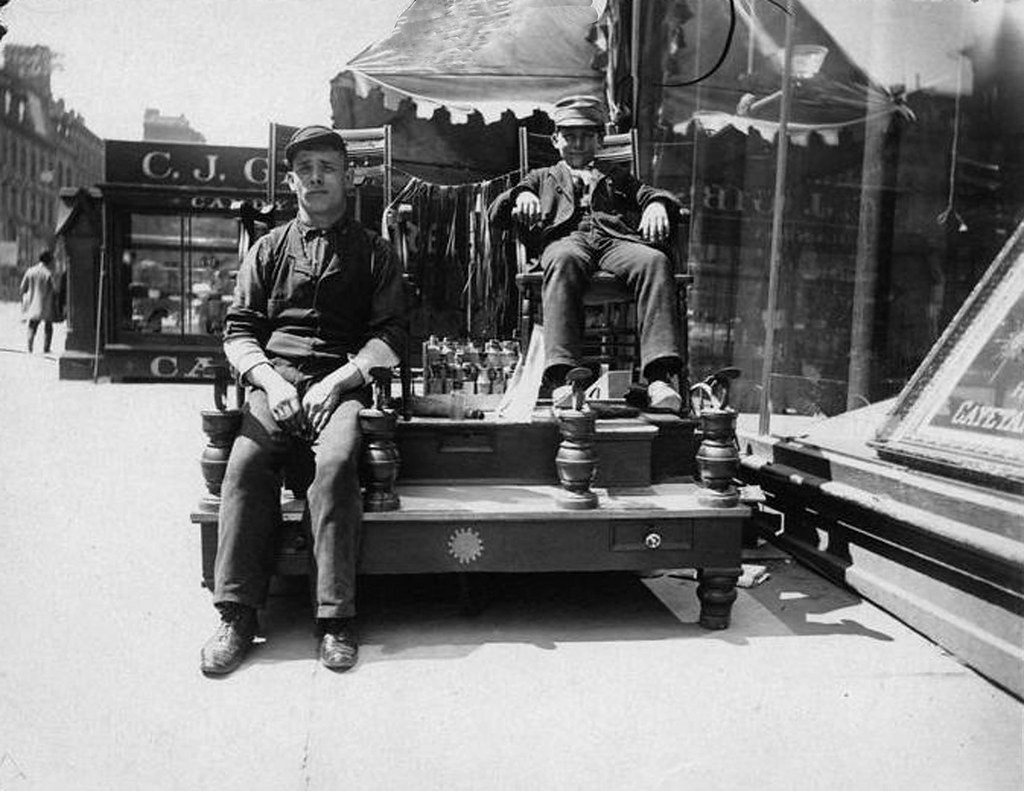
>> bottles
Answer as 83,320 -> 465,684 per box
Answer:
423,334 -> 524,396
449,381 -> 466,420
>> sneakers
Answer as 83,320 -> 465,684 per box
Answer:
201,607 -> 259,674
320,619 -> 357,667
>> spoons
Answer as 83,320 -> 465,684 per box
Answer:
369,367 -> 391,409
715,368 -> 742,410
567,366 -> 593,412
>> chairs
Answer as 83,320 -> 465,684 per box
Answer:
238,121 -> 418,418
509,173 -> 692,389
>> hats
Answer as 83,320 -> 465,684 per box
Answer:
549,95 -> 605,127
284,126 -> 345,167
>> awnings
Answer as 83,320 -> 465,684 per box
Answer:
664,0 -> 902,143
330,0 -> 602,130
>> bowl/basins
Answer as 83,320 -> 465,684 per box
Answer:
778,44 -> 829,79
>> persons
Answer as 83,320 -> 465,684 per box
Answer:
486,96 -> 683,412
18,252 -> 59,352
199,126 -> 423,675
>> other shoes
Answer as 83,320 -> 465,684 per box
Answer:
640,374 -> 682,411
551,379 -> 583,410
